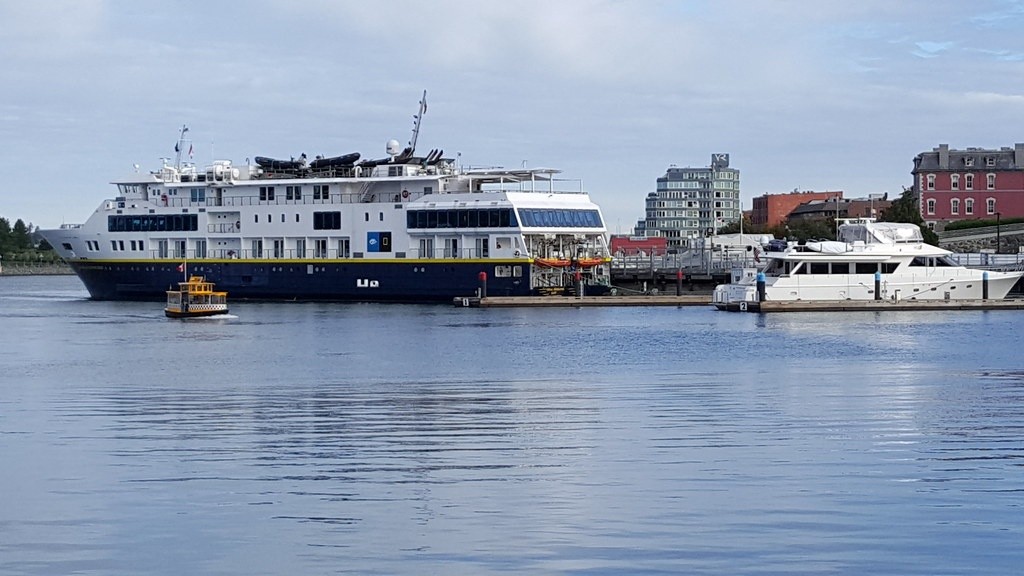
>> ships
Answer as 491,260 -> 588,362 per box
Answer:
39,90 -> 615,304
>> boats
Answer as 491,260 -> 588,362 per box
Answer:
164,258 -> 229,319
710,241 -> 1024,310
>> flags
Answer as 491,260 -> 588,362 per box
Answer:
175,262 -> 184,272
188,144 -> 194,159
753,247 -> 761,263
175,142 -> 179,152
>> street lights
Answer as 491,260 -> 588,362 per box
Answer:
994,211 -> 1002,254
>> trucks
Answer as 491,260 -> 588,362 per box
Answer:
606,234 -> 667,257
839,224 -> 924,243
708,243 -> 755,268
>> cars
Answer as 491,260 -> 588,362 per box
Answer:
769,239 -> 785,251
